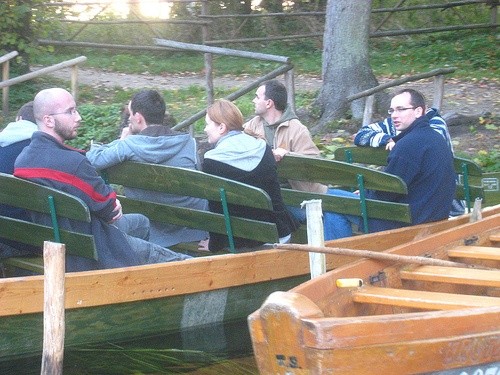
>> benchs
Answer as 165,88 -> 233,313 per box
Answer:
0,146 -> 485,278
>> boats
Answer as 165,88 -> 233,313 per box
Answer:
248,213 -> 500,375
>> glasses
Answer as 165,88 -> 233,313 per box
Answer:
49,109 -> 76,117
388,107 -> 416,115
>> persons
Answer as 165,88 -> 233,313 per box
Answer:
352,92 -> 467,217
116,99 -> 179,140
1,100 -> 40,256
12,87 -> 195,269
326,88 -> 457,241
239,77 -> 329,226
82,91 -> 213,251
198,96 -> 301,255
